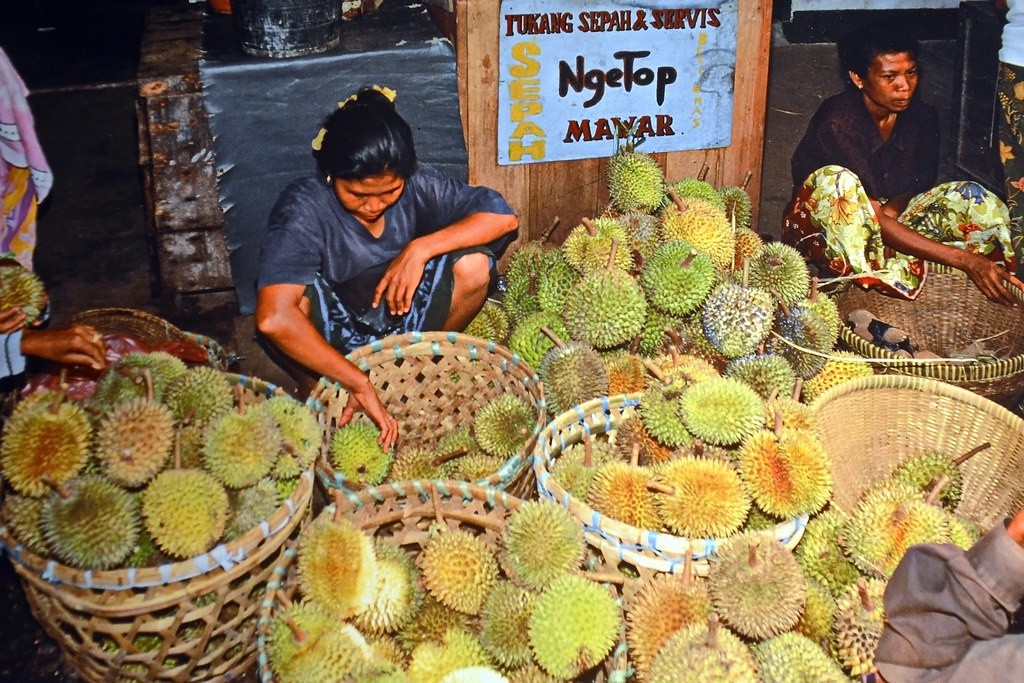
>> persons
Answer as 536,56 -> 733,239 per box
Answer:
256,84 -> 518,453
874,505 -> 1024,683
0,47 -> 104,378
782,34 -> 1024,308
998,0 -> 1024,281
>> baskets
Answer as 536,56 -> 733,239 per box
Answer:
0,259 -> 1024,683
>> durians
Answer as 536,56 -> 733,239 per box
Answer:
0,263 -> 322,683
265,150 -> 982,683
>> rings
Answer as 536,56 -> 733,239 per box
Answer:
387,413 -> 394,420
92,334 -> 101,342
